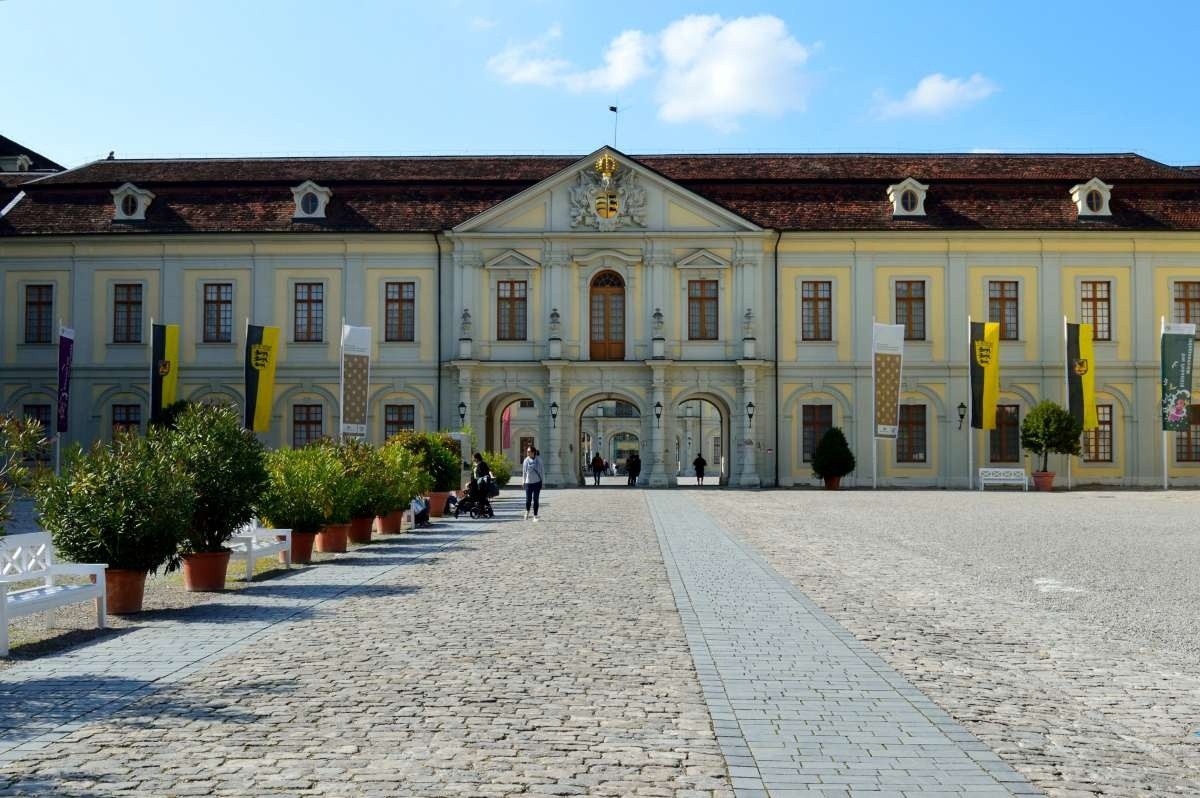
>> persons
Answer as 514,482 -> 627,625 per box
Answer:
626,453 -> 636,485
522,446 -> 543,522
414,496 -> 434,527
693,453 -> 707,485
458,481 -> 474,511
611,460 -> 618,477
591,452 -> 603,487
447,489 -> 460,514
603,460 -> 609,476
472,453 -> 494,519
631,455 -> 641,486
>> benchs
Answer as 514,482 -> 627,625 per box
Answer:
0,530 -> 111,654
978,468 -> 1031,491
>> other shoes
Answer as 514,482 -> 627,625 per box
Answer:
533,514 -> 540,522
488,515 -> 496,519
420,521 -> 435,528
524,510 -> 531,520
475,514 -> 483,518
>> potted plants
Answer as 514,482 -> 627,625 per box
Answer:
30,387 -> 516,631
807,423 -> 857,491
1015,397 -> 1084,491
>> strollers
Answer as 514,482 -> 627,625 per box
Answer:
456,475 -> 500,519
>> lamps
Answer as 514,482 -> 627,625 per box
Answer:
547,401 -> 562,431
653,399 -> 663,430
746,402 -> 759,429
955,401 -> 969,430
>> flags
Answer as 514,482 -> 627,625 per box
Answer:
969,321 -> 1000,430
1067,324 -> 1099,430
57,326 -> 75,432
341,325 -> 371,440
244,325 -> 281,435
872,323 -> 905,439
1160,323 -> 1196,431
149,324 -> 181,415
